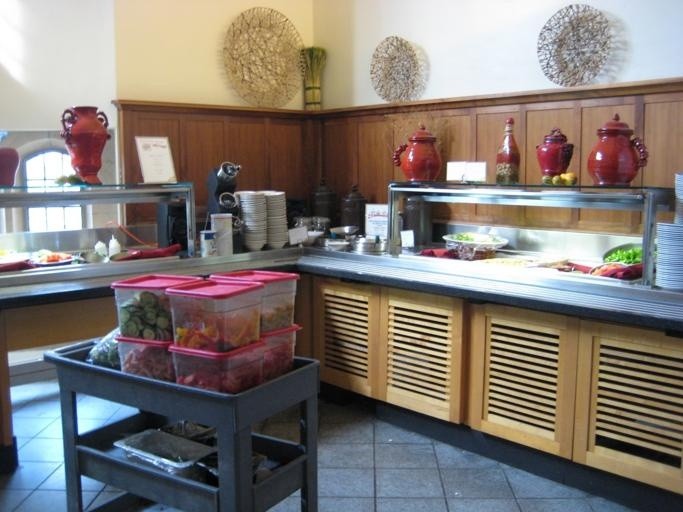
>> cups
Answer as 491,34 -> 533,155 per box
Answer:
304,231 -> 324,245
199,213 -> 233,257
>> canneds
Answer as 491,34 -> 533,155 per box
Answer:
294,217 -> 330,247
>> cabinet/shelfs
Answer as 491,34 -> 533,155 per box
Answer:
46,340 -> 321,512
309,273 -> 682,512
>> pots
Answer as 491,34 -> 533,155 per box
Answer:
351,234 -> 388,256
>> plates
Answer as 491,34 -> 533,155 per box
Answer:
654,173 -> 683,292
30,253 -> 78,265
1,252 -> 32,269
442,232 -> 510,254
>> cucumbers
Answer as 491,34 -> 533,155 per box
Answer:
115,291 -> 173,340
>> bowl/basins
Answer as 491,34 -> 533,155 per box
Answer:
325,241 -> 349,252
234,191 -> 288,250
329,225 -> 360,239
589,243 -> 657,281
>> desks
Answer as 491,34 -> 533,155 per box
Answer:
1,276 -> 120,475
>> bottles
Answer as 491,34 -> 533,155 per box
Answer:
314,217 -> 330,245
295,219 -> 311,245
404,197 -> 425,251
94,241 -> 106,256
311,179 -> 336,220
340,183 -> 364,234
109,234 -> 120,258
